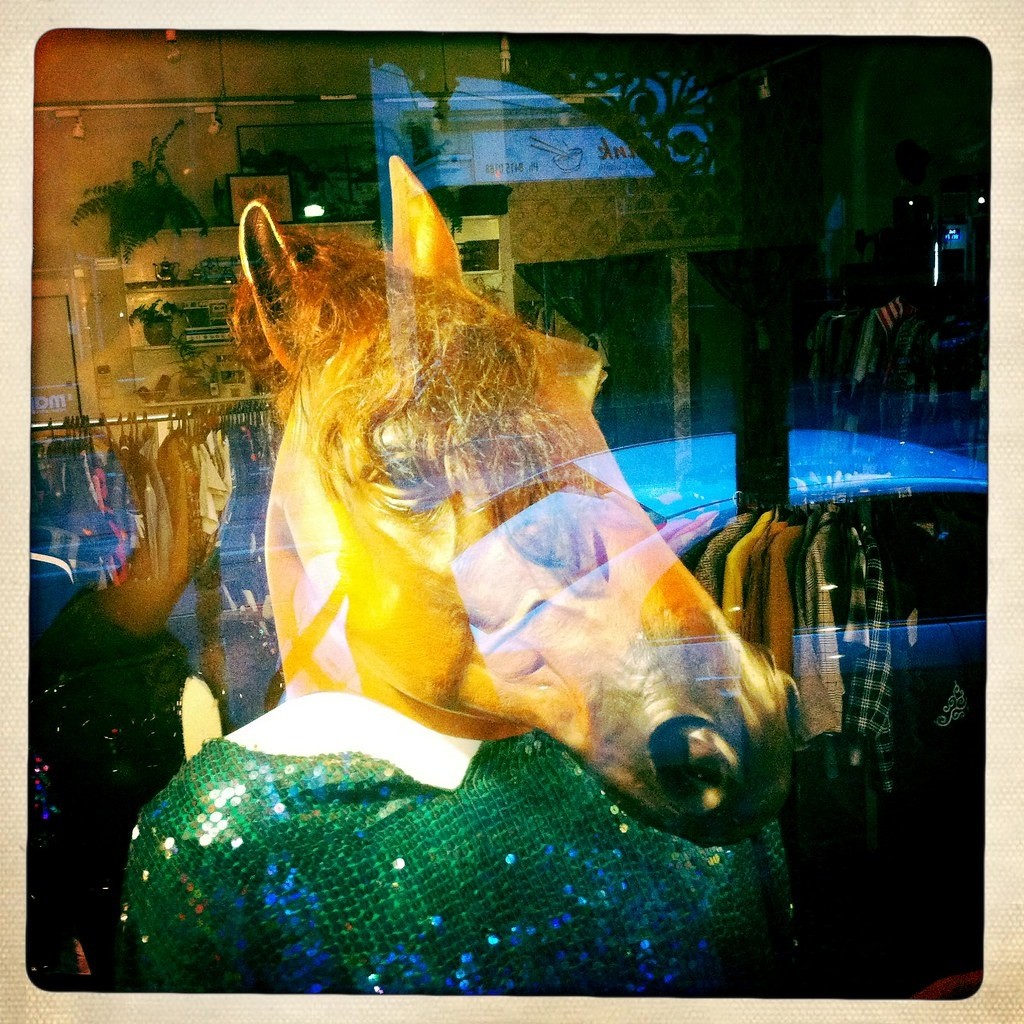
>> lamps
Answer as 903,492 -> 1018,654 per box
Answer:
72,115 -> 84,139
206,113 -> 222,137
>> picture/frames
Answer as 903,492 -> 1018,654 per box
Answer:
226,172 -> 297,226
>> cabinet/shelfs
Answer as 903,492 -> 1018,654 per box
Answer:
120,215 -> 505,409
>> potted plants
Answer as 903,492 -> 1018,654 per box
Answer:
127,298 -> 184,345
167,332 -> 211,399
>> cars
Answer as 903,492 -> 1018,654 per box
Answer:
293,428 -> 986,747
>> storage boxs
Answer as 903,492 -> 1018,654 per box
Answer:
460,184 -> 514,216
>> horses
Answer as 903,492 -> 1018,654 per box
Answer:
237,154 -> 802,846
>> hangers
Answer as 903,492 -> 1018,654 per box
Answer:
728,473 -> 913,508
47,400 -> 280,453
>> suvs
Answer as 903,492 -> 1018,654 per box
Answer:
28,412 -> 575,729
